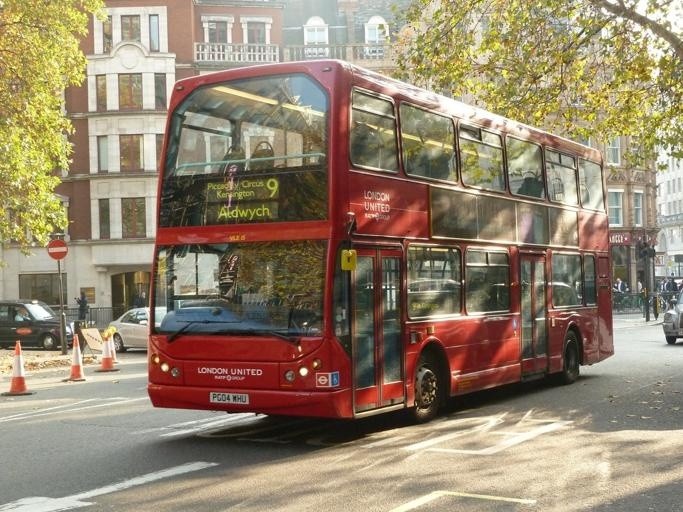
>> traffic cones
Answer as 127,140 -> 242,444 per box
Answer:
66,334 -> 86,381
0,340 -> 38,396
94,328 -> 121,372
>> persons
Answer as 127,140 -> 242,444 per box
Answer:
13,307 -> 30,322
73,291 -> 89,320
612,271 -> 683,312
216,264 -> 261,306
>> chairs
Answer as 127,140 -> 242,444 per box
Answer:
352,122 -> 590,209
217,141 -> 274,173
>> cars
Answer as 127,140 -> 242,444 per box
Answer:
662,285 -> 683,344
106,306 -> 167,353
0,298 -> 74,351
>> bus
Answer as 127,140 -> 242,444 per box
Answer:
143,59 -> 615,425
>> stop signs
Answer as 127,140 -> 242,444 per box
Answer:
46,238 -> 69,355
